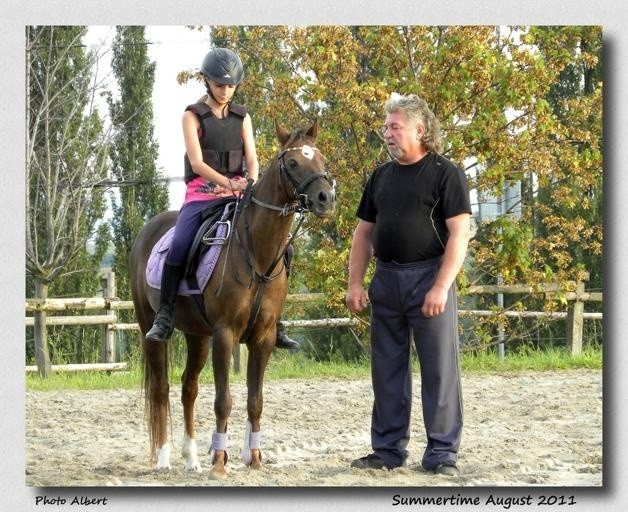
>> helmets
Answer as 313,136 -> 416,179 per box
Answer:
200,47 -> 244,84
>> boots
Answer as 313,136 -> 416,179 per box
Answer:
276,321 -> 300,348
144,263 -> 186,342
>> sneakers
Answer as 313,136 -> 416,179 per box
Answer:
433,459 -> 457,476
352,453 -> 389,469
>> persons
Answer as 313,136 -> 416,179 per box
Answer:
143,47 -> 303,351
344,94 -> 473,478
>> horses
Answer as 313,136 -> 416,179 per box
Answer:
127,116 -> 339,481
